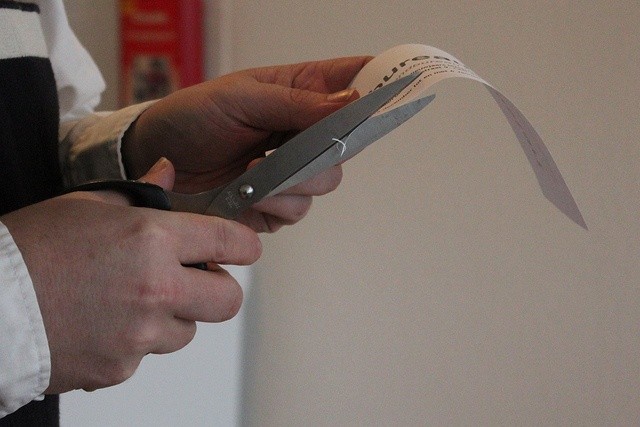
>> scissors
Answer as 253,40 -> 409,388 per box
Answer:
66,72 -> 436,271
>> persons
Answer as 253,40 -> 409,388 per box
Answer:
0,0 -> 378,427
